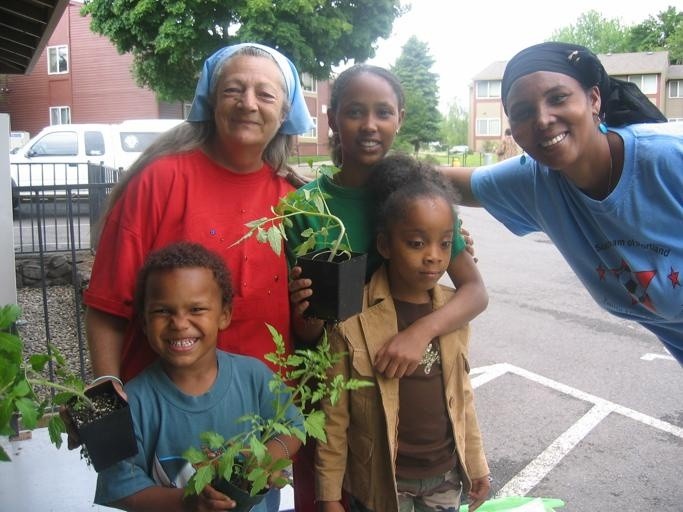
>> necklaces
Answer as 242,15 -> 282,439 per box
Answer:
606,153 -> 613,194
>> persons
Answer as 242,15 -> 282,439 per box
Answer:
496,128 -> 517,163
307,151 -> 493,512
59,41 -> 479,430
89,239 -> 306,511
282,61 -> 492,512
427,39 -> 683,376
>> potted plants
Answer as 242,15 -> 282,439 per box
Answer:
0,304 -> 140,474
174,323 -> 374,512
225,156 -> 367,319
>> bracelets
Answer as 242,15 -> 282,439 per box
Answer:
272,435 -> 291,461
90,374 -> 123,385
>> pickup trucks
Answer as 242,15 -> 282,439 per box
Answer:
8,118 -> 188,210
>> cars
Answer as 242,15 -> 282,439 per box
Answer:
448,145 -> 473,156
